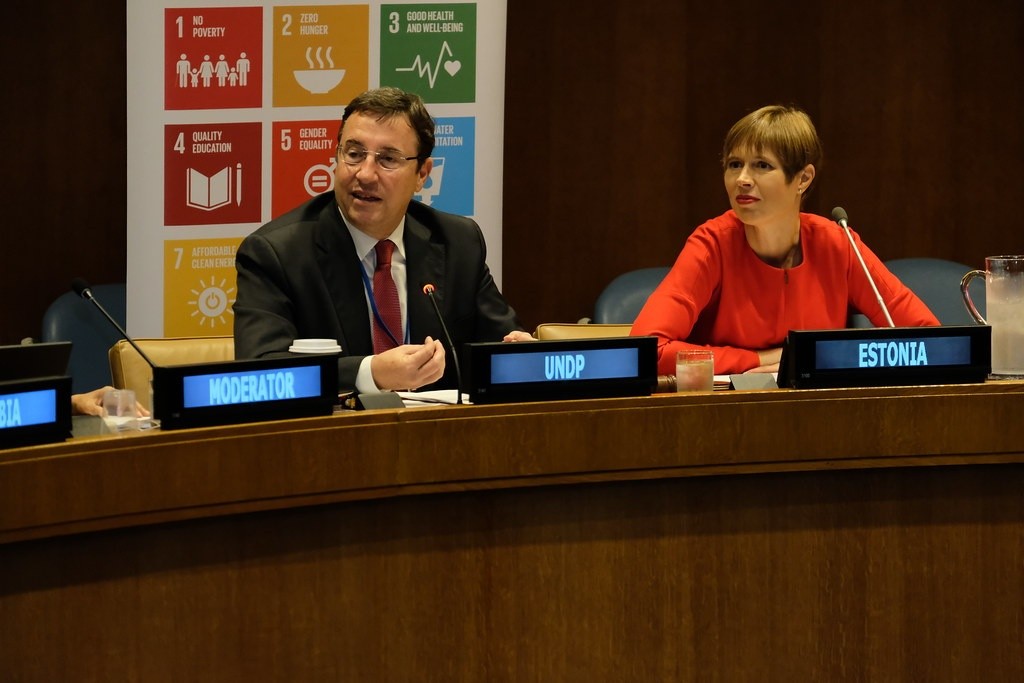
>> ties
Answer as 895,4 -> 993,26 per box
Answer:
374,239 -> 403,355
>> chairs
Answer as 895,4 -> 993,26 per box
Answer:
850,257 -> 987,329
107,335 -> 237,422
43,284 -> 128,396
593,266 -> 704,349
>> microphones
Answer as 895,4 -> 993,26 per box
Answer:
831,206 -> 895,327
70,276 -> 159,368
421,281 -> 464,405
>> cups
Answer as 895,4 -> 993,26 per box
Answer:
959,255 -> 1024,381
288,339 -> 343,415
676,350 -> 714,392
100,390 -> 138,435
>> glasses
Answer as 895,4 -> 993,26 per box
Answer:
336,141 -> 419,171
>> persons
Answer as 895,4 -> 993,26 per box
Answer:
71,385 -> 150,418
629,105 -> 940,375
229,87 -> 538,393
